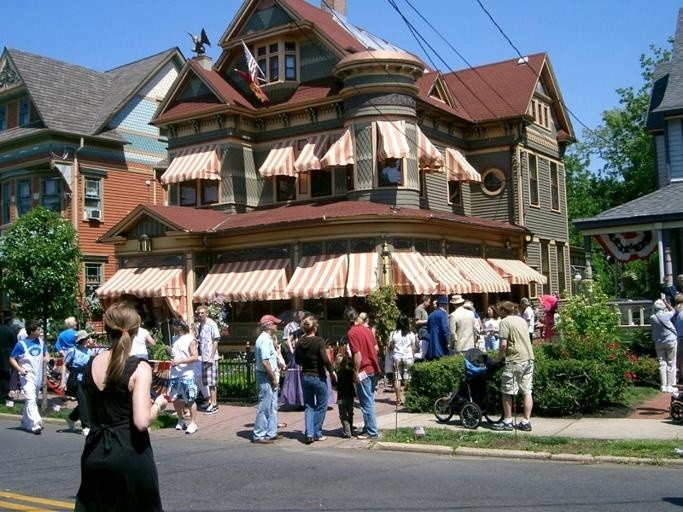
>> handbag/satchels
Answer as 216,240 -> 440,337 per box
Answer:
389,340 -> 395,349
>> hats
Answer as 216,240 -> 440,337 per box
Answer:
521,297 -> 530,306
450,295 -> 465,304
75,331 -> 90,343
260,314 -> 282,325
435,296 -> 448,304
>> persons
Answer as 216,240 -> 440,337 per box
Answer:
655,273 -> 682,307
647,298 -> 678,394
1,310 -> 94,435
73,299 -> 169,511
253,294 -> 558,444
129,305 -> 220,434
670,291 -> 683,391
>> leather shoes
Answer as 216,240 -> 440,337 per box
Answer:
253,434 -> 283,444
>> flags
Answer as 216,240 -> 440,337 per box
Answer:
235,68 -> 270,104
241,41 -> 259,83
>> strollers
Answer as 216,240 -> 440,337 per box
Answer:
668,380 -> 682,424
432,347 -> 505,429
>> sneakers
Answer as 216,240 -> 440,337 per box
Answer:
175,418 -> 197,433
514,422 -> 532,431
65,416 -> 76,431
491,421 -> 514,431
306,436 -> 328,445
20,417 -> 41,433
200,403 -> 218,415
342,428 -> 378,439
82,427 -> 90,435
661,385 -> 679,393
